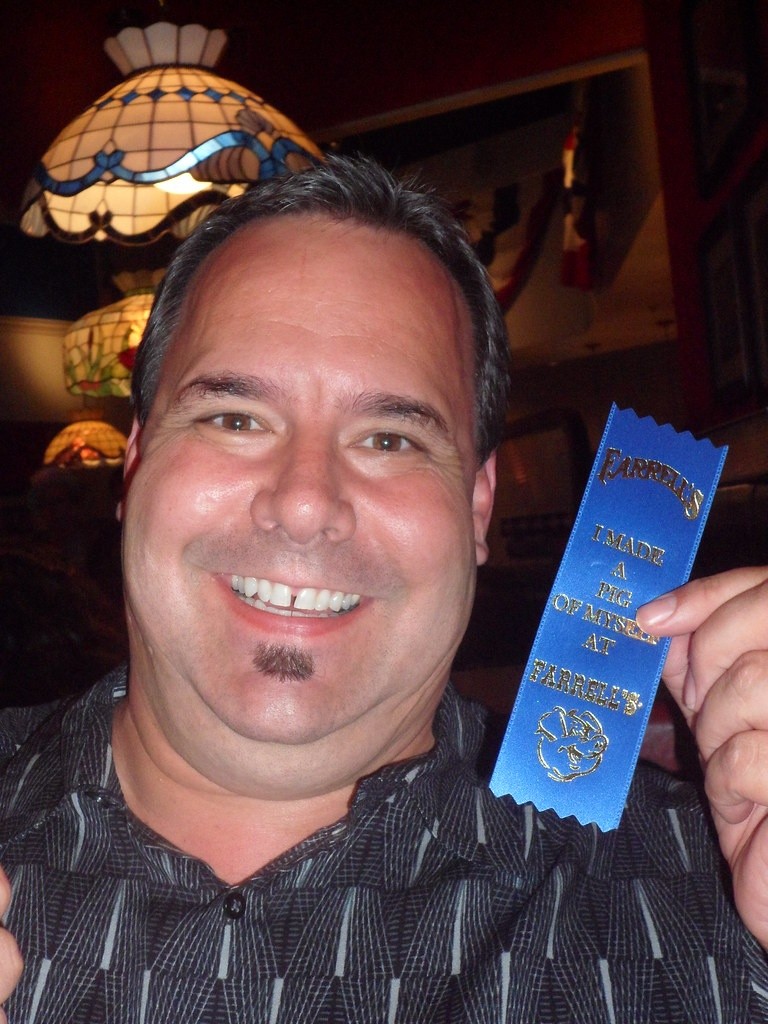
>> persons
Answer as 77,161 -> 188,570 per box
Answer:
0,152 -> 768,1024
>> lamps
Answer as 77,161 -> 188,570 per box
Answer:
16,0 -> 327,247
63,268 -> 169,396
42,409 -> 126,468
